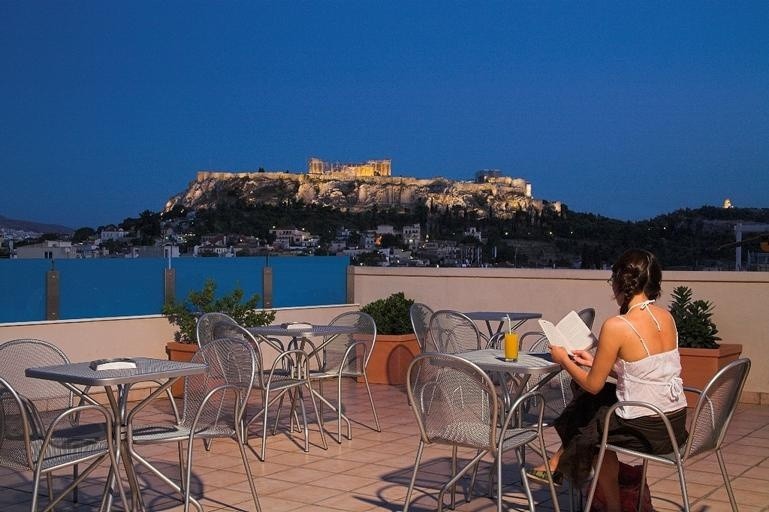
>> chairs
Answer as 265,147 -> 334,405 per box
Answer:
0,339 -> 115,512
403,354 -> 562,512
0,378 -> 132,512
132,338 -> 261,512
409,303 -> 597,468
196,312 -> 382,462
584,358 -> 750,512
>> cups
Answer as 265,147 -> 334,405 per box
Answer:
504,331 -> 518,362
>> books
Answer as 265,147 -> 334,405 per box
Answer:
281,322 -> 313,329
537,309 -> 599,358
91,357 -> 137,370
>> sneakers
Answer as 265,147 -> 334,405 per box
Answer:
525,469 -> 562,488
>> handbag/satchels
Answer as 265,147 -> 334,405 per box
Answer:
586,462 -> 652,512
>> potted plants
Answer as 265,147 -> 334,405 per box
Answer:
667,284 -> 742,408
161,279 -> 276,401
352,291 -> 427,385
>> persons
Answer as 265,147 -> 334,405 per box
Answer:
523,246 -> 689,512
